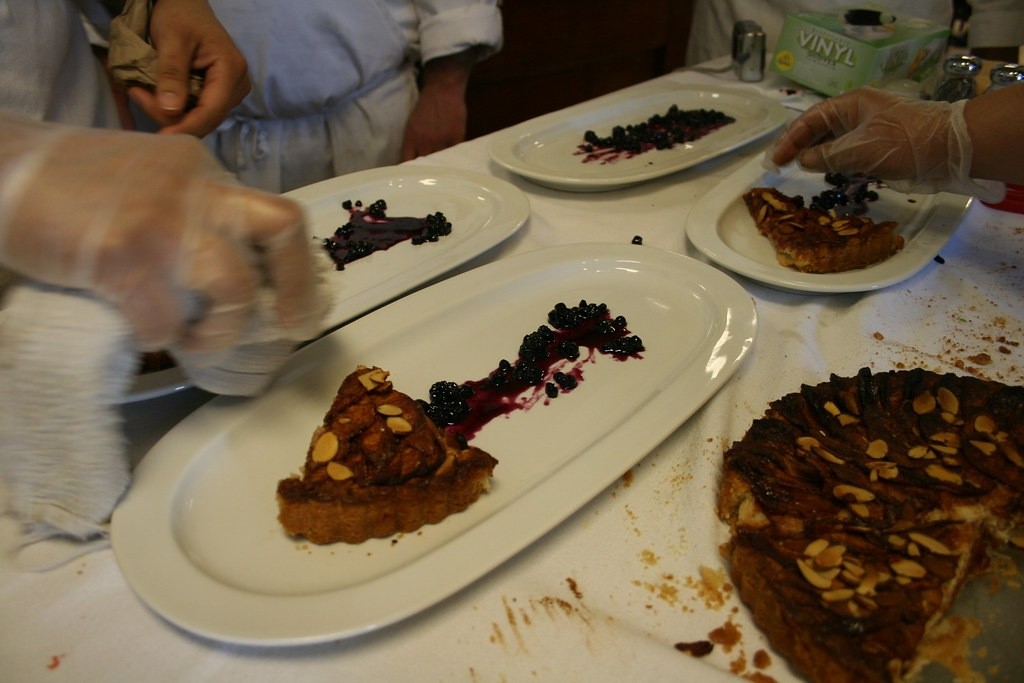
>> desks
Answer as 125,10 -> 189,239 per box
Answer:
2,53 -> 1024,682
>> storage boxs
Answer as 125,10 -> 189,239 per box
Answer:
770,5 -> 951,99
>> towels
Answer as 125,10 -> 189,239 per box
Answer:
0,282 -> 138,543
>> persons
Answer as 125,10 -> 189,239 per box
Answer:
771,79 -> 1024,176
196,0 -> 505,194
1,0 -> 323,400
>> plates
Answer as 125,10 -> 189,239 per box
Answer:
486,85 -> 788,193
684,152 -> 974,293
108,243 -> 760,648
99,163 -> 530,404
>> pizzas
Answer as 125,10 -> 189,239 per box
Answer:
722,364 -> 1024,683
743,186 -> 904,273
276,362 -> 499,544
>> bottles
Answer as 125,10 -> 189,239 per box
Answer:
982,64 -> 1024,95
933,56 -> 982,104
